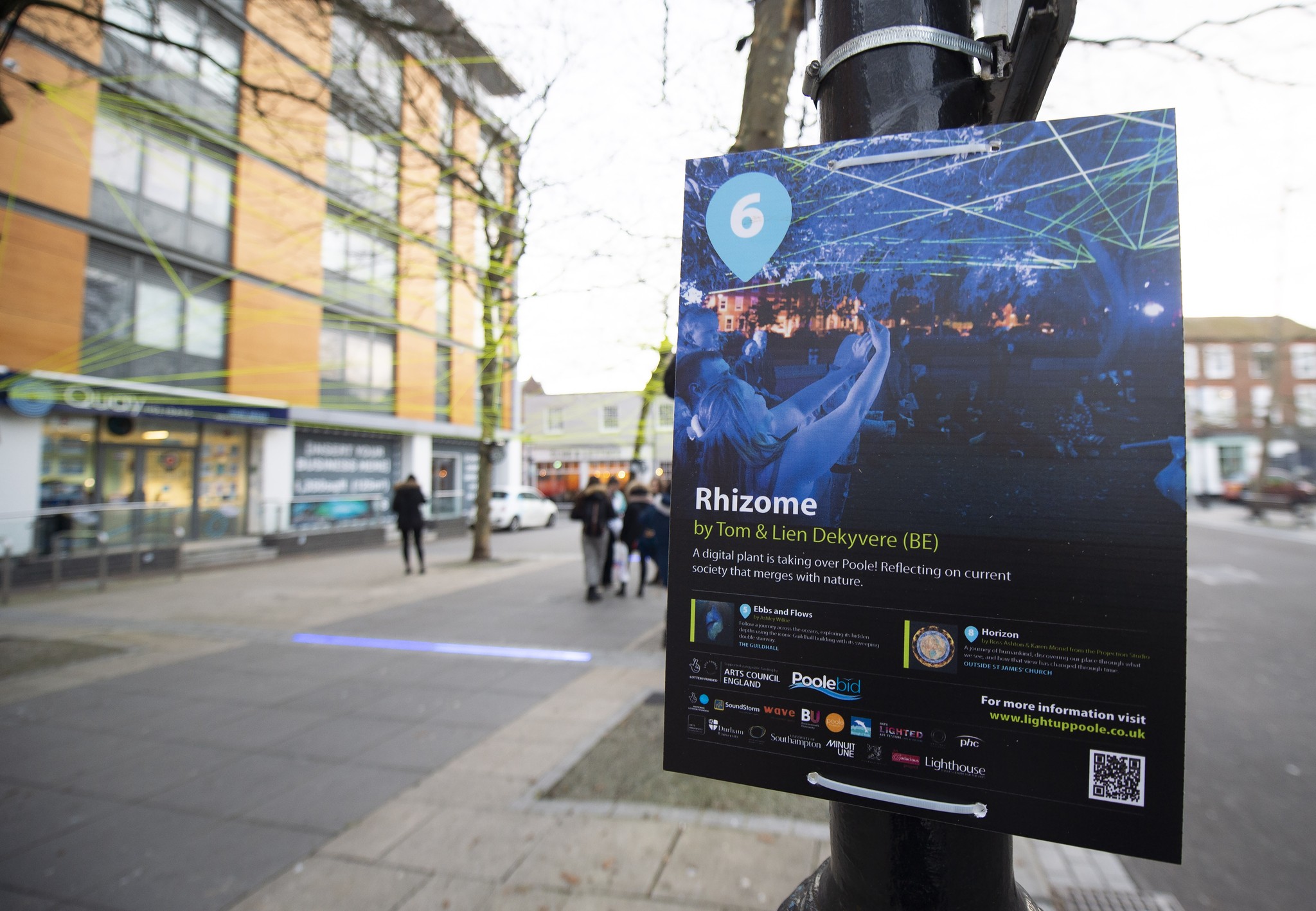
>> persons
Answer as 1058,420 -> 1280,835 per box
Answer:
1119,436 -> 1187,514
392,474 -> 427,575
571,473 -> 670,603
679,299 -> 915,526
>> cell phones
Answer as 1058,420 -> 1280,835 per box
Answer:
859,305 -> 871,324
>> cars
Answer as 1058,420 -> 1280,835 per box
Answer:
465,482 -> 560,533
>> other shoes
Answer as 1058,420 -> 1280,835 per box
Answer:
586,588 -> 601,601
616,588 -> 625,598
637,589 -> 645,597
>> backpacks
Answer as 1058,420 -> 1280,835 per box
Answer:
581,500 -> 608,540
